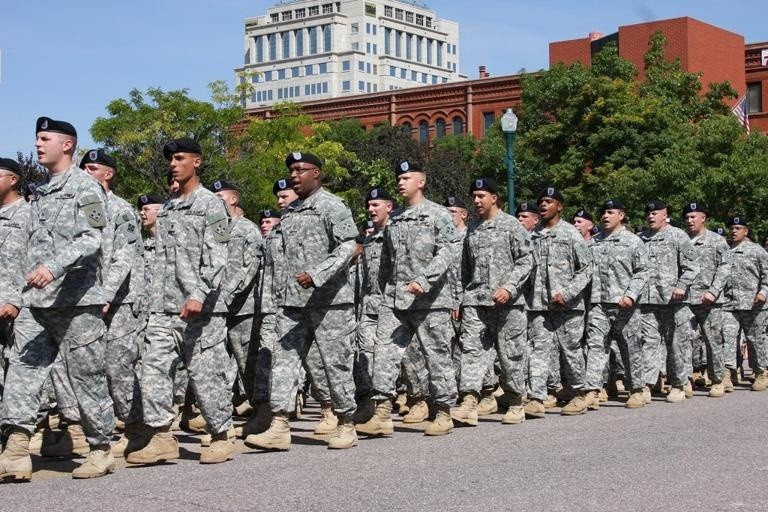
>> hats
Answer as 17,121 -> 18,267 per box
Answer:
259,209 -> 281,223
273,178 -> 293,195
285,152 -> 322,168
210,181 -> 239,192
515,187 -> 563,214
35,117 -> 77,136
443,177 -> 496,209
163,139 -> 201,158
0,158 -> 23,176
395,158 -> 423,177
574,200 -> 625,221
366,188 -> 391,201
645,201 -> 709,217
714,217 -> 747,235
137,192 -> 162,209
79,150 -> 115,168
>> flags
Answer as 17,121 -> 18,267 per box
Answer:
730,98 -> 753,135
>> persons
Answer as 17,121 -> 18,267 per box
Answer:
243,143 -> 362,455
0,149 -> 339,458
354,185 -> 430,423
124,140 -> 246,465
353,154 -> 460,436
3,115 -> 123,480
443,177 -> 768,427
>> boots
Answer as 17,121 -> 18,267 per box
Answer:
115,393 -> 304,464
395,379 -> 625,436
0,413 -> 115,484
313,394 -> 394,449
625,366 -> 768,409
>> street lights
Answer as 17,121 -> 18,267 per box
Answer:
502,108 -> 519,216
